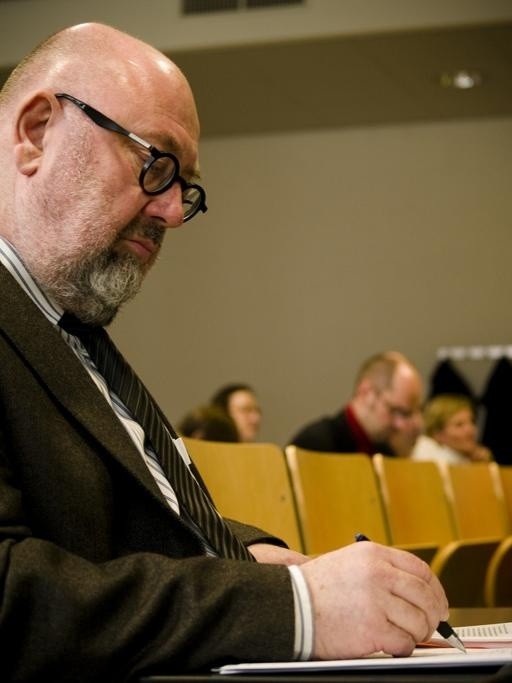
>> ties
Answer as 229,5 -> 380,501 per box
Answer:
57,308 -> 257,563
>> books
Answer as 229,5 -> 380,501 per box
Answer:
413,618 -> 511,649
216,644 -> 512,677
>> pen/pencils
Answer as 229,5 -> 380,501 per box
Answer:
353,530 -> 467,651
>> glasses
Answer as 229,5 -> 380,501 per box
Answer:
54,93 -> 208,222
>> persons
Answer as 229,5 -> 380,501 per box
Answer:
410,391 -> 494,465
286,347 -> 422,456
173,406 -> 244,442
385,397 -> 426,456
210,378 -> 260,442
0,17 -> 454,680
